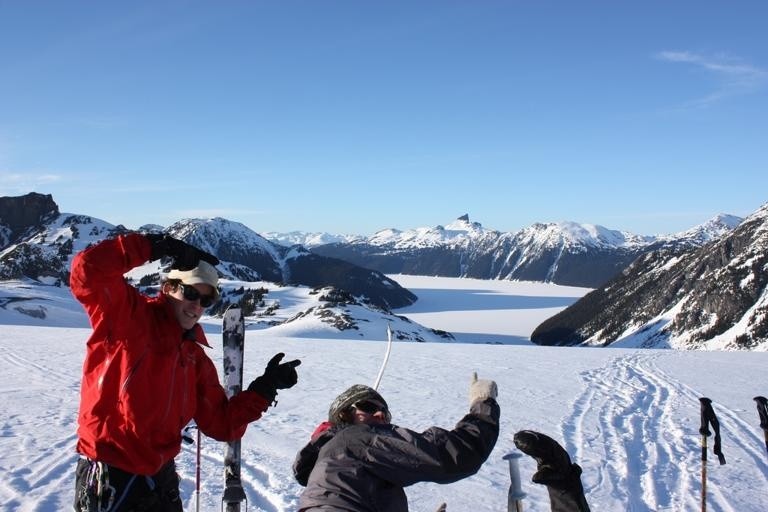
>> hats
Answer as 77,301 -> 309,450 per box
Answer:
328,384 -> 388,426
158,260 -> 220,295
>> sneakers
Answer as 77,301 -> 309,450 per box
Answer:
512,430 -> 573,472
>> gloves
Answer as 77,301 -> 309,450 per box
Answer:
248,352 -> 300,402
469,370 -> 500,407
146,231 -> 219,272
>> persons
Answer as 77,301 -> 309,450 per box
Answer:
294,368 -> 502,511
513,426 -> 594,510
68,231 -> 302,511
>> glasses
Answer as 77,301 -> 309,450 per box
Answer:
347,399 -> 386,419
176,281 -> 215,307
